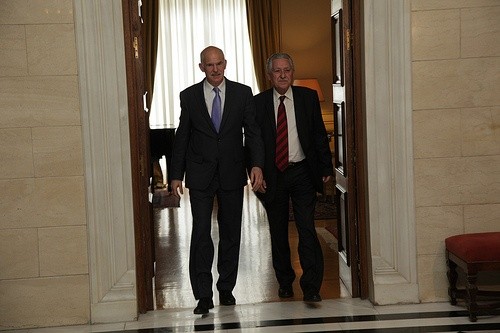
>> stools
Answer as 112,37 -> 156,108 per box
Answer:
444,232 -> 500,323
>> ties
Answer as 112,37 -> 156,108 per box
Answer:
211,88 -> 221,133
275,96 -> 289,172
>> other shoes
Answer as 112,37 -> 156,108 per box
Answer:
193,297 -> 214,314
278,287 -> 294,298
219,291 -> 236,306
303,294 -> 321,302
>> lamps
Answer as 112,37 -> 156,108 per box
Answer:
293,79 -> 324,102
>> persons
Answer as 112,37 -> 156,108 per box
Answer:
170,46 -> 253,313
244,53 -> 333,302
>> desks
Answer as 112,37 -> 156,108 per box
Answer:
150,124 -> 176,194
